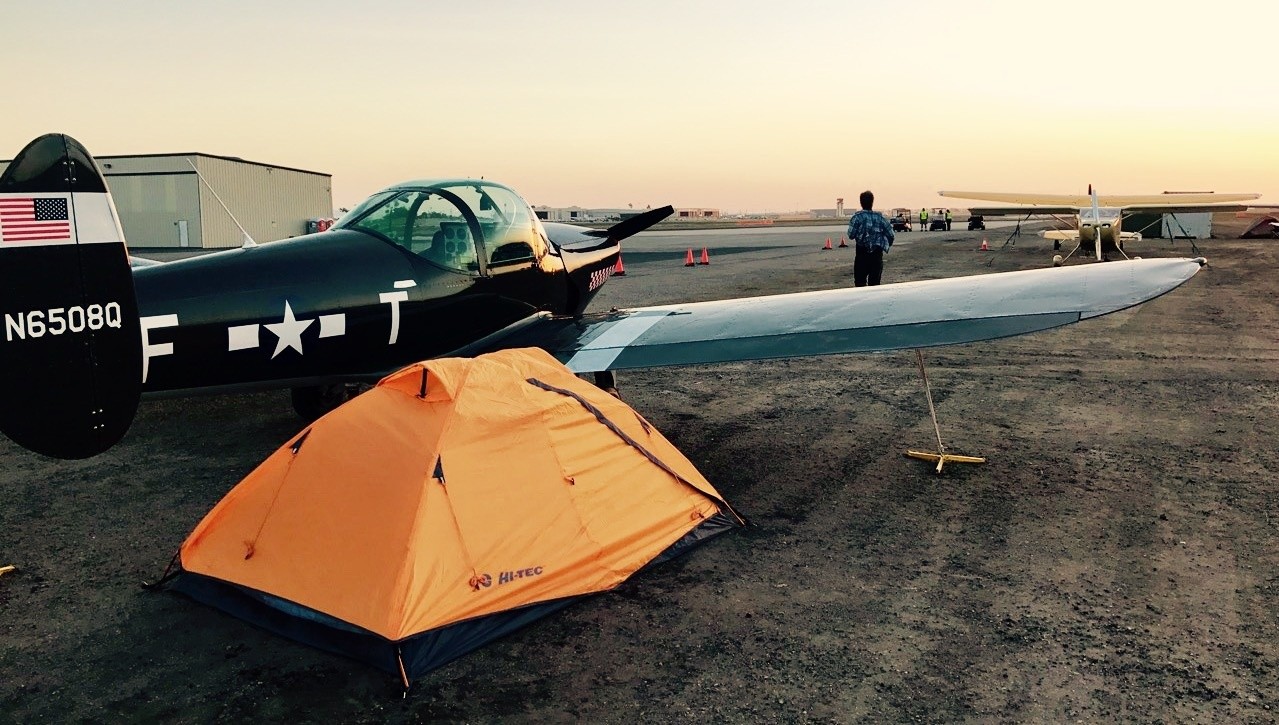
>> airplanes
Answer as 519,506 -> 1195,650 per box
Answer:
936,180 -> 1279,264
0,131 -> 1211,462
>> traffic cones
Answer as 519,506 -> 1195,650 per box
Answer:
979,238 -> 989,251
838,234 -> 848,247
822,236 -> 833,250
612,254 -> 626,277
698,245 -> 710,265
684,247 -> 696,267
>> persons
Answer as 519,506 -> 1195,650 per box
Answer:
919,208 -> 928,231
945,209 -> 953,231
938,210 -> 943,215
847,191 -> 894,288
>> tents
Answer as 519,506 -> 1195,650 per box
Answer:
144,346 -> 747,706
